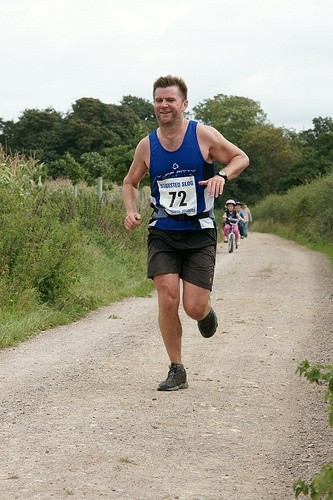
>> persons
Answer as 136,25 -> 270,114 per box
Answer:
223,199 -> 252,248
123,75 -> 250,392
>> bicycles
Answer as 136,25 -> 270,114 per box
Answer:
224,217 -> 242,253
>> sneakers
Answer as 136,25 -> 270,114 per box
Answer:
157,362 -> 188,391
197,307 -> 218,338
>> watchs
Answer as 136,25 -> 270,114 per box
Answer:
216,171 -> 227,182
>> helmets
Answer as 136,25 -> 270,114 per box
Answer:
226,199 -> 236,206
235,201 -> 241,205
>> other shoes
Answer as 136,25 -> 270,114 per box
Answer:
240,236 -> 244,239
236,240 -> 240,246
224,237 -> 228,242
